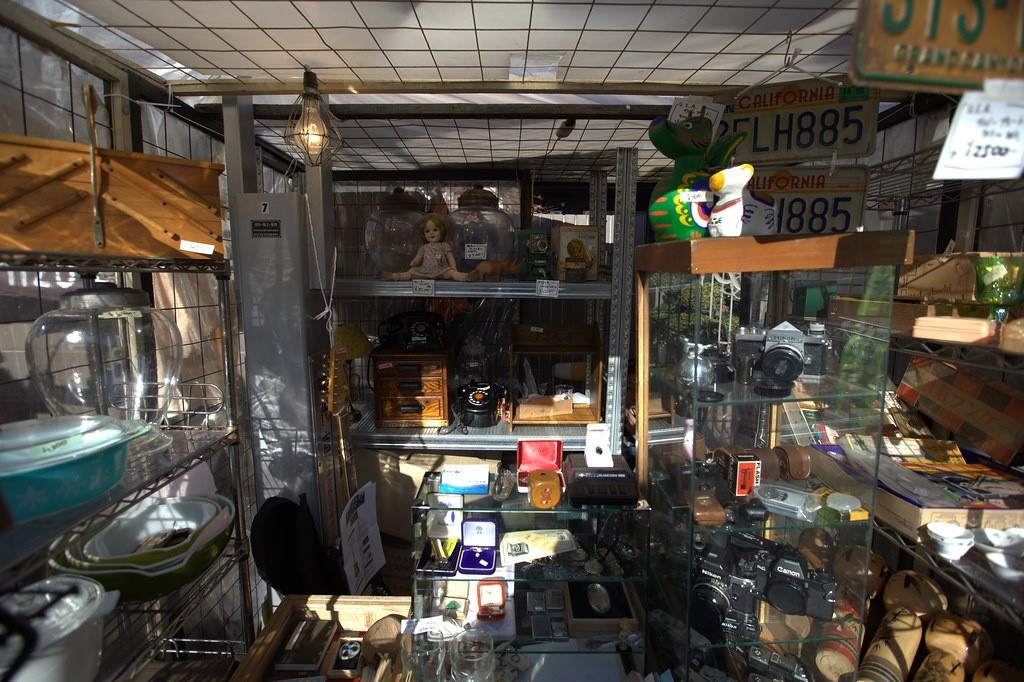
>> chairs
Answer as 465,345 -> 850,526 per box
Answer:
251,496 -> 392,596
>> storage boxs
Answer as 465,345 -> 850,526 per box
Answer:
501,491 -> 535,531
584,424 -> 614,467
805,444 -> 1024,543
477,579 -> 506,621
564,580 -> 639,640
458,518 -> 499,575
441,464 -> 489,494
230,594 -> 414,682
727,454 -> 762,497
440,580 -> 469,620
352,449 -> 502,540
415,492 -> 464,577
549,225 -> 599,281
516,438 -> 566,493
515,394 -> 573,419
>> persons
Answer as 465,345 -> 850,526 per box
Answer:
383,213 -> 478,282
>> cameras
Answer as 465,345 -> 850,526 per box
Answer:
646,322 -> 840,682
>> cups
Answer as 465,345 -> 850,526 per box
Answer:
400,629 -> 446,682
450,628 -> 495,682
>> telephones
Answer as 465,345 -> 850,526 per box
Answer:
455,380 -> 507,428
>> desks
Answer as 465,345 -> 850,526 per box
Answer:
512,640 -> 629,682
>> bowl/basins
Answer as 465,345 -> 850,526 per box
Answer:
926,522 -> 1024,585
48,493 -> 236,604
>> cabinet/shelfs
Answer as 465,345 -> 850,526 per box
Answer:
641,366 -> 675,424
411,473 -> 651,655
0,250 -> 255,682
634,229 -> 916,682
505,320 -> 603,432
807,314 -> 1024,631
370,348 -> 450,429
332,148 -> 637,452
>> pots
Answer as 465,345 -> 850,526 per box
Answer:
0,573 -> 121,682
